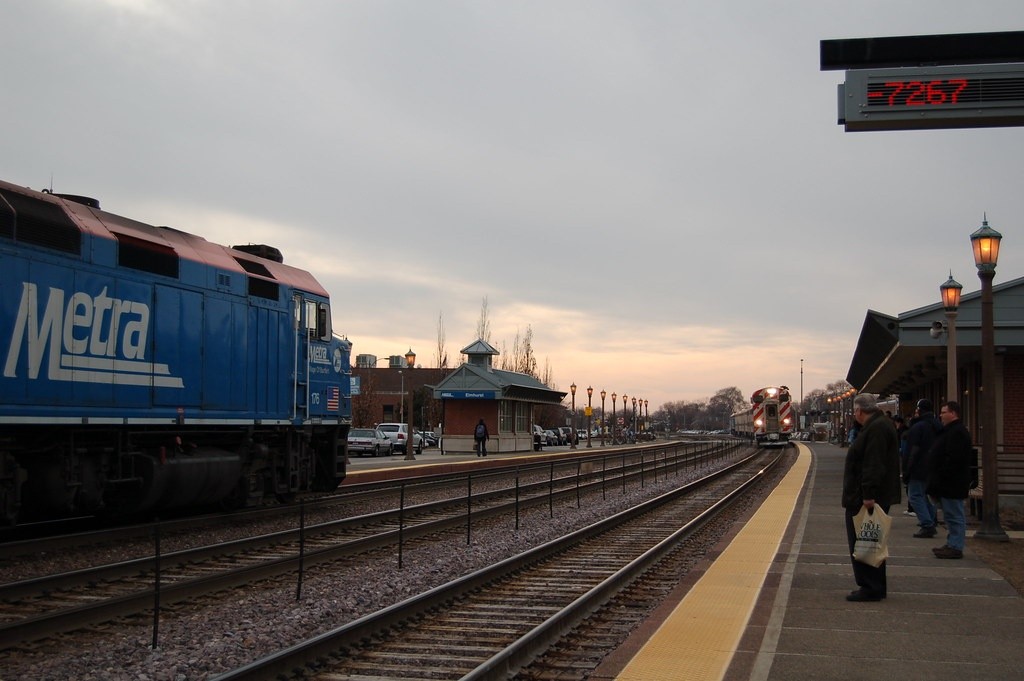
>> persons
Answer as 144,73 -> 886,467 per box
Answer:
474,417 -> 489,457
842,393 -> 901,600
880,399 -> 942,537
927,401 -> 973,561
840,423 -> 854,448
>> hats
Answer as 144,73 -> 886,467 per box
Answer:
915,400 -> 933,414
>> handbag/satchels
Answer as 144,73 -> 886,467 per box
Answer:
852,503 -> 892,568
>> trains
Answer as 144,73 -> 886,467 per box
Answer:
0,176 -> 357,538
729,385 -> 794,447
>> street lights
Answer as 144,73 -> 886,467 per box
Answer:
800,358 -> 807,439
637,398 -> 642,442
400,347 -> 419,460
569,382 -> 577,450
586,385 -> 595,448
630,396 -> 637,444
610,391 -> 618,445
644,399 -> 650,442
622,394 -> 628,444
967,209 -> 1012,542
939,268 -> 963,404
398,370 -> 405,424
599,388 -> 607,447
367,357 -> 394,424
826,387 -> 859,448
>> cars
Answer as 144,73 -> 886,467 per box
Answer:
347,428 -> 395,457
561,426 -> 609,445
543,430 -> 559,446
418,429 -> 438,447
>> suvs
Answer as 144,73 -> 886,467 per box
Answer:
550,427 -> 568,446
376,423 -> 423,454
534,425 -> 547,451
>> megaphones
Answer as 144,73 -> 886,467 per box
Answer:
930,319 -> 947,339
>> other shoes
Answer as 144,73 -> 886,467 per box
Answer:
932,544 -> 963,560
904,510 -> 918,517
847,587 -> 887,601
913,526 -> 937,538
